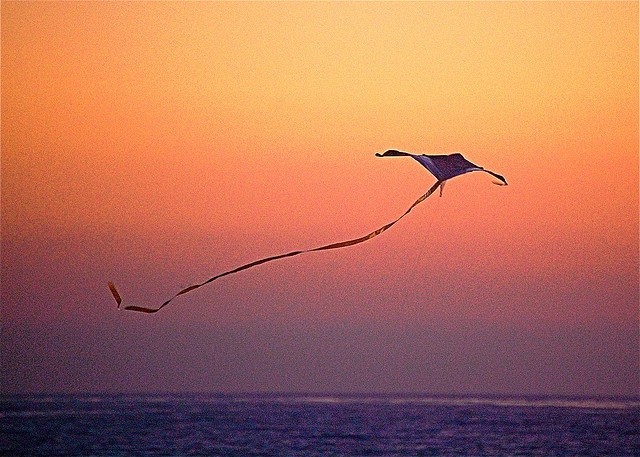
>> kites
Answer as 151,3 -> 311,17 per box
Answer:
107,149 -> 509,314
